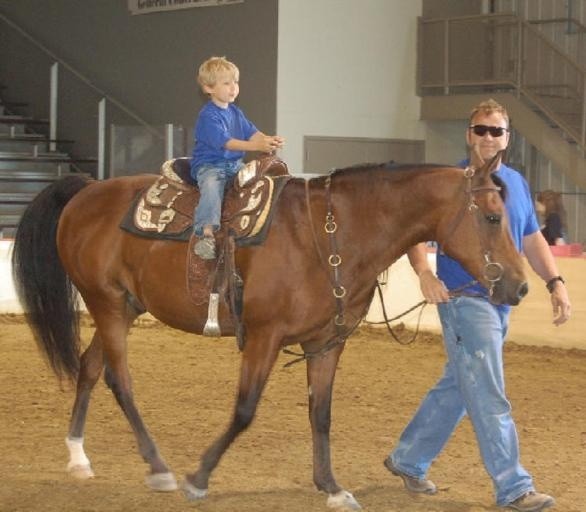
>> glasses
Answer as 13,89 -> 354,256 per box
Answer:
469,124 -> 510,137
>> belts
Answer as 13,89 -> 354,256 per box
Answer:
448,291 -> 485,297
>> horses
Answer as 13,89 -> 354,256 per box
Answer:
10,150 -> 532,511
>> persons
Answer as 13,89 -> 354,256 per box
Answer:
382,98 -> 572,512
534,189 -> 570,246
189,56 -> 286,260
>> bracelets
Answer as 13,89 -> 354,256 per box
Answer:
546,276 -> 565,294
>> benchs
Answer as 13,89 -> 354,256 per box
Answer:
0,82 -> 98,242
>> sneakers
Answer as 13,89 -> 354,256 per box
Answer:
505,489 -> 556,512
384,456 -> 436,494
194,235 -> 217,260
